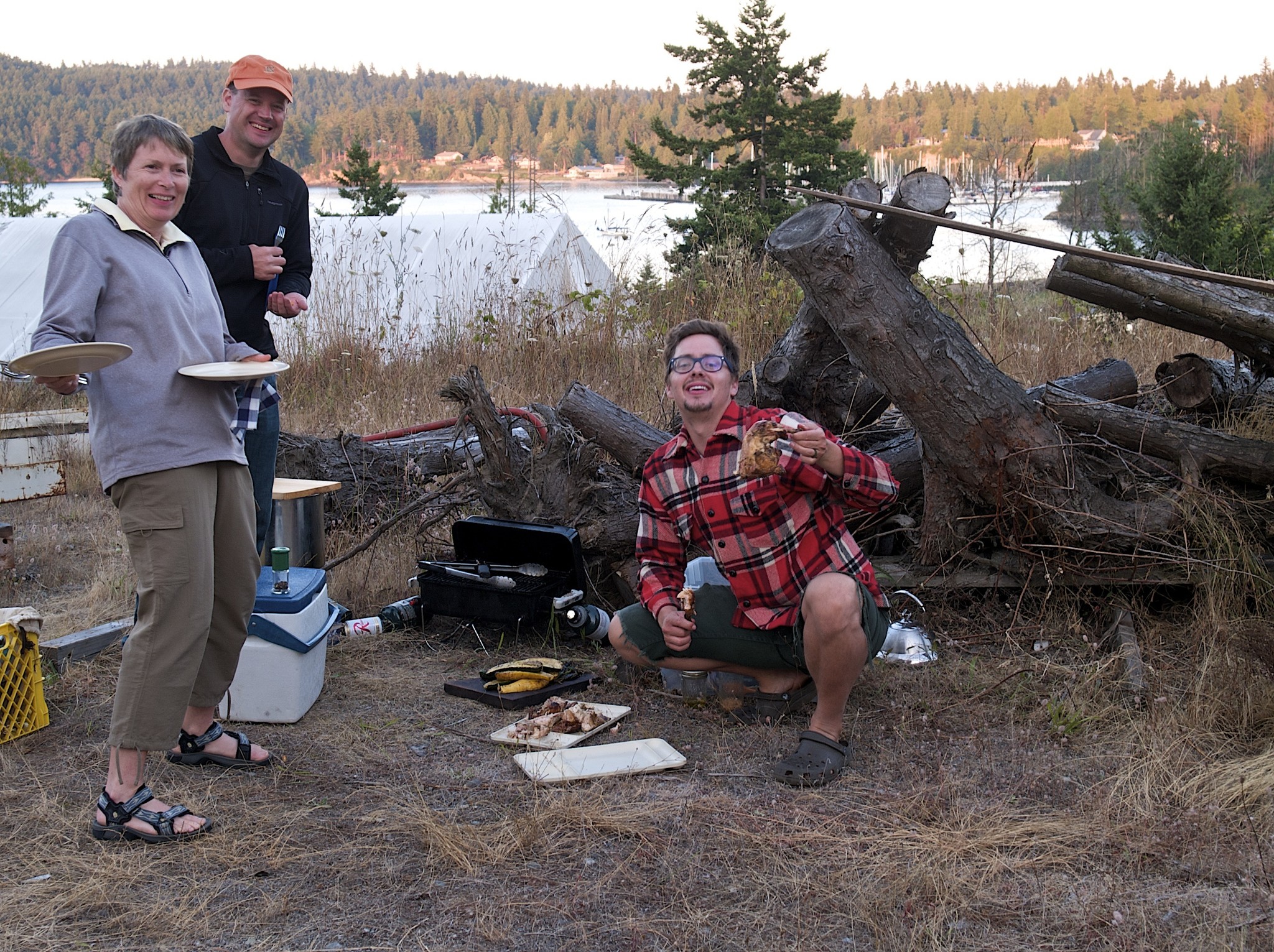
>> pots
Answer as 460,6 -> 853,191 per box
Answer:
270,493 -> 327,569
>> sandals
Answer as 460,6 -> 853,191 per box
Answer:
167,720 -> 274,768
93,783 -> 212,841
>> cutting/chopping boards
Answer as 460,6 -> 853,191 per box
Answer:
442,670 -> 601,710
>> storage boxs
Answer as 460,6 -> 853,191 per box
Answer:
0,607 -> 50,745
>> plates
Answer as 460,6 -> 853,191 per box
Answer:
514,738 -> 687,785
179,360 -> 288,382
490,699 -> 631,750
9,342 -> 133,377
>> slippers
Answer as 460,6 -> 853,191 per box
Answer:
774,730 -> 852,786
729,677 -> 818,725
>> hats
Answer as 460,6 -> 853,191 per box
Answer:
225,55 -> 293,103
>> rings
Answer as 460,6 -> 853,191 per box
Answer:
811,449 -> 818,459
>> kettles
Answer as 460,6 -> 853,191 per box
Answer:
878,590 -> 938,665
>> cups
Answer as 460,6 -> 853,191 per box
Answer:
717,670 -> 744,712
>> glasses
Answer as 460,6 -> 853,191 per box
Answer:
667,355 -> 734,374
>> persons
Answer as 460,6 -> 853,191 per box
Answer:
22,114 -> 273,841
168,54 -> 316,570
634,318 -> 902,787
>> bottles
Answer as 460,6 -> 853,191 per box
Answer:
567,605 -> 612,647
381,596 -> 434,633
